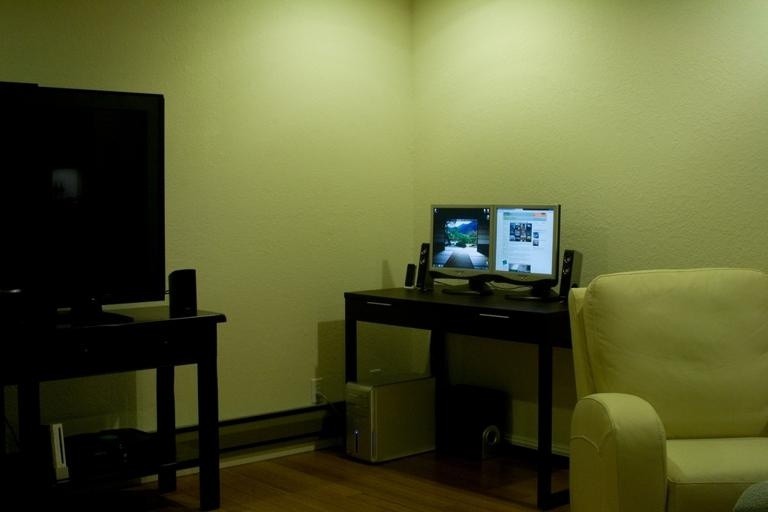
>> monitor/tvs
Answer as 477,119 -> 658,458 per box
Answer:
428,204 -> 494,294
0,82 -> 165,327
493,204 -> 561,303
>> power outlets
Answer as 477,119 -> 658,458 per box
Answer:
312,377 -> 324,404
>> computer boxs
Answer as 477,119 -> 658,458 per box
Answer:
345,371 -> 438,463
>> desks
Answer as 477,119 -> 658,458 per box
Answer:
0,301 -> 226,512
343,286 -> 575,512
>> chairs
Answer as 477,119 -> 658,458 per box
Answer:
567,268 -> 768,512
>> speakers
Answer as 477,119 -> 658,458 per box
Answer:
168,269 -> 197,318
559,250 -> 582,303
416,242 -> 432,292
